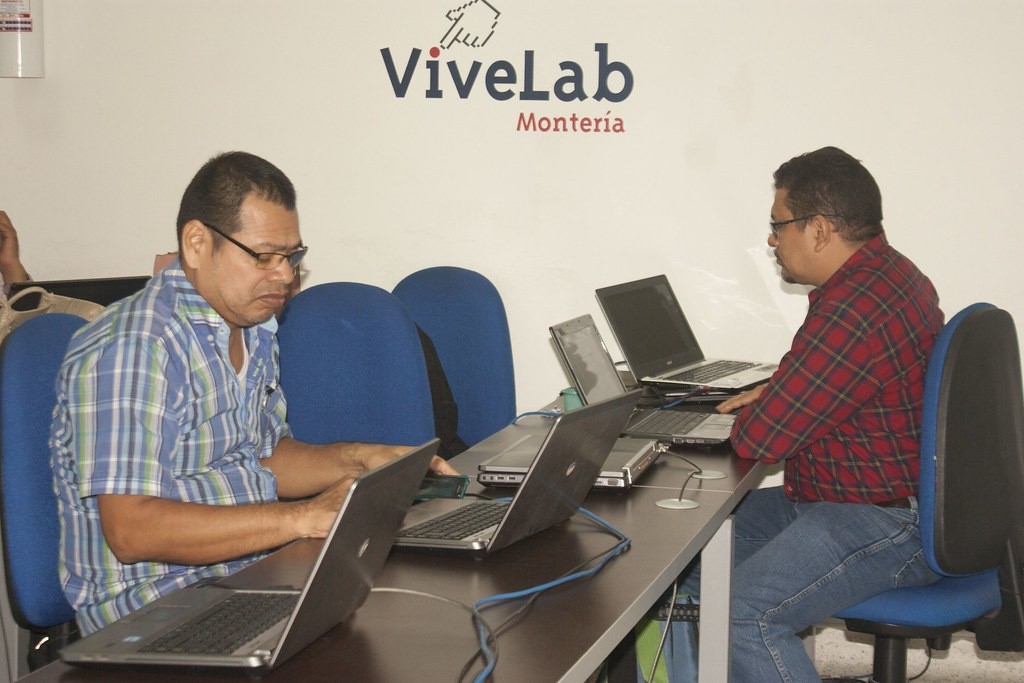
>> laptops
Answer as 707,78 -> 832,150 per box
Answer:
548,314 -> 737,445
59,438 -> 441,679
478,433 -> 662,491
594,274 -> 781,392
394,388 -> 645,559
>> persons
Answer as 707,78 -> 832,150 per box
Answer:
661,144 -> 948,679
47,149 -> 458,641
1,208 -> 42,314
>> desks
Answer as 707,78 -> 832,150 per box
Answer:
14,367 -> 786,683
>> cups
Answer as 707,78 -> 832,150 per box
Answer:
562,387 -> 584,412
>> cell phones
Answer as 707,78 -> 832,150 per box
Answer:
413,474 -> 470,502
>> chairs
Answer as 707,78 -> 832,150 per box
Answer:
817,302 -> 1022,683
279,283 -> 442,498
4,311 -> 99,672
391,265 -> 519,469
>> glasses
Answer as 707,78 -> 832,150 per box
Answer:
200,222 -> 308,270
769,214 -> 845,232
